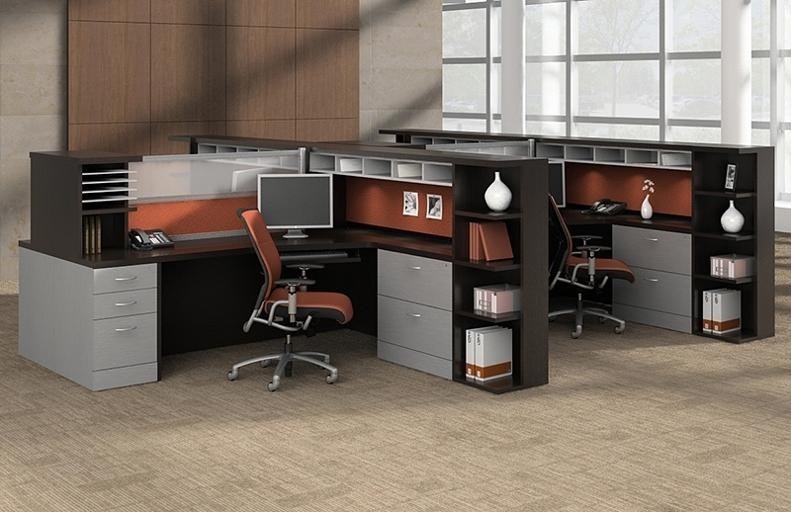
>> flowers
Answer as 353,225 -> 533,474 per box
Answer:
641,179 -> 656,195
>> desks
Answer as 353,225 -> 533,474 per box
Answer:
547,204 -> 692,334
127,225 -> 453,382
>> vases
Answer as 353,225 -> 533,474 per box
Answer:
721,199 -> 745,234
640,195 -> 654,219
484,171 -> 513,213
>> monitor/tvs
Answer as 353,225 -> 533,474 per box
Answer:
257,174 -> 333,238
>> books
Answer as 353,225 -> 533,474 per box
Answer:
82,215 -> 103,256
458,219 -> 521,313
708,253 -> 758,277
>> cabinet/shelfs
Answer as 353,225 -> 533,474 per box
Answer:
453,158 -> 549,395
16,148 -> 161,390
691,145 -> 776,344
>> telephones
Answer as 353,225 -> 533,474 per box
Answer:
129,228 -> 175,249
591,199 -> 627,216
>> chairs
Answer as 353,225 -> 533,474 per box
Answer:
227,206 -> 355,392
548,192 -> 636,339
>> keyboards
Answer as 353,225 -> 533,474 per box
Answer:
278,250 -> 348,260
